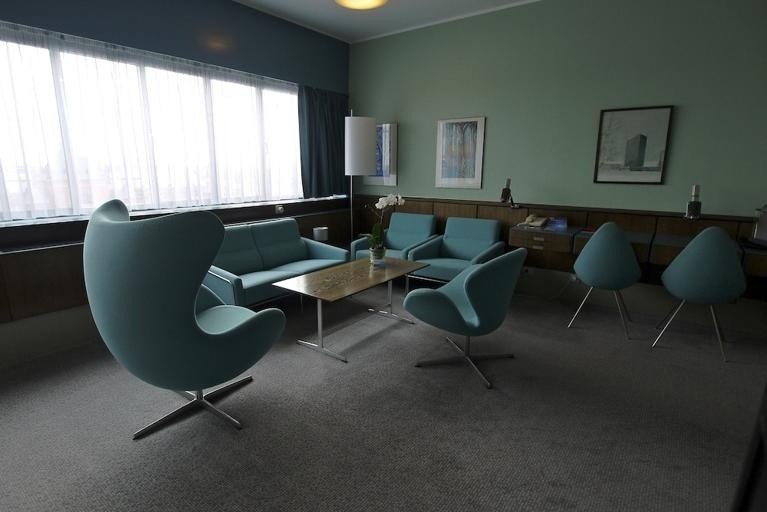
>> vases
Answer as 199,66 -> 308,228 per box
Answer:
370,249 -> 385,267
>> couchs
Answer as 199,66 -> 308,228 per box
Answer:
202,215 -> 350,305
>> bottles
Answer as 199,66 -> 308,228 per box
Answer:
686,184 -> 702,218
500,178 -> 511,203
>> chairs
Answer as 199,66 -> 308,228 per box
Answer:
404,216 -> 502,297
83,199 -> 287,440
351,211 -> 438,261
651,226 -> 747,363
567,221 -> 642,340
403,246 -> 529,389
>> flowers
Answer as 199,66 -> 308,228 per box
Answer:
368,193 -> 406,249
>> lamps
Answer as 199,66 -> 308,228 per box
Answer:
343,109 -> 377,240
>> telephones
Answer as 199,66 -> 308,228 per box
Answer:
517,214 -> 547,228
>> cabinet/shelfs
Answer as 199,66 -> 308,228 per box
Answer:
509,217 -> 572,254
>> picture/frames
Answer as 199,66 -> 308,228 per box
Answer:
434,115 -> 487,190
592,104 -> 675,186
